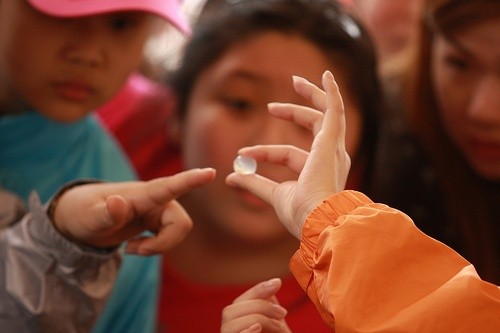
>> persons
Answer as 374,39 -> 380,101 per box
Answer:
220,70 -> 500,333
1,2 -> 500,333
248,0 -> 250,1
0,168 -> 216,333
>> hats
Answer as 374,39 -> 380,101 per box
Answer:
29,0 -> 193,36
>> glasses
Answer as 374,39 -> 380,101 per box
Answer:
324,5 -> 363,42
